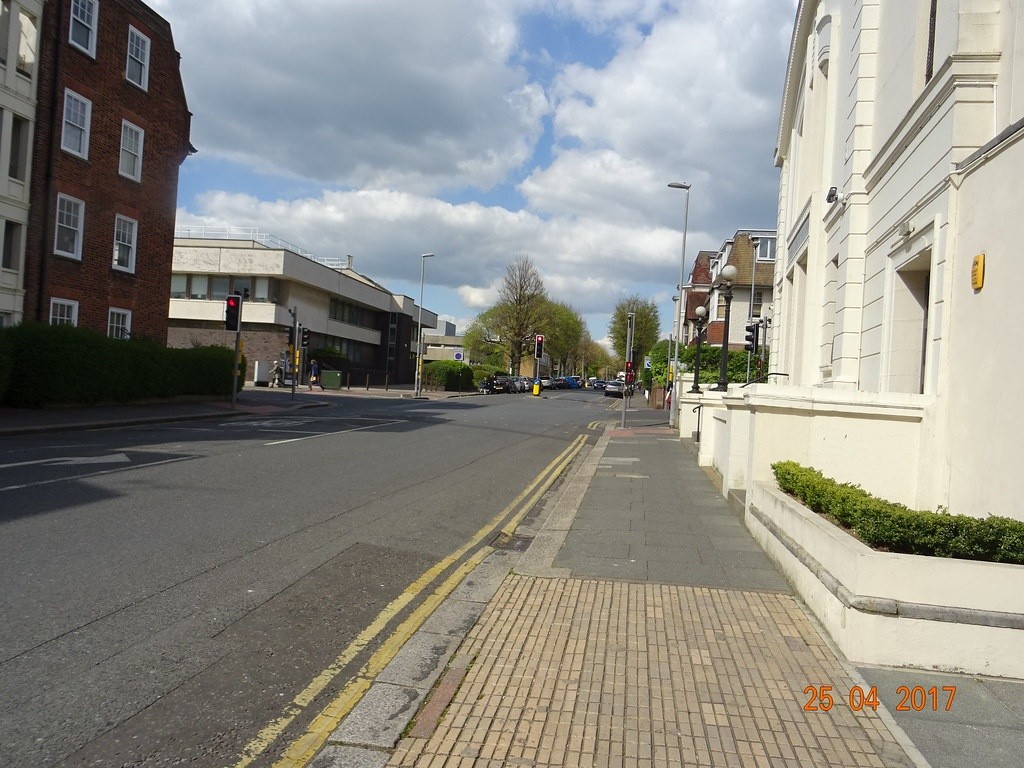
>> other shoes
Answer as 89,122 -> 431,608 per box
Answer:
270,385 -> 273,388
323,387 -> 325,391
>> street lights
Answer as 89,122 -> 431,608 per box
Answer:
707,264 -> 737,392
626,312 -> 636,408
414,251 -> 435,396
687,304 -> 707,395
666,180 -> 692,428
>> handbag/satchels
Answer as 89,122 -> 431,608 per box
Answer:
310,376 -> 316,382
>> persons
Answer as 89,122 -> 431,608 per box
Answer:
268,361 -> 286,388
308,359 -> 325,391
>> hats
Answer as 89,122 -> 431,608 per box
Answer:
274,361 -> 278,364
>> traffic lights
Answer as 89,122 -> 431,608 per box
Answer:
745,324 -> 759,355
534,335 -> 544,359
284,326 -> 295,345
225,294 -> 242,332
300,327 -> 310,349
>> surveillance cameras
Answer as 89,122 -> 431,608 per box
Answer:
825,187 -> 837,203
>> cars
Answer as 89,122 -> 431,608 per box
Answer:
521,376 -> 534,393
532,371 -> 638,399
510,375 -> 526,393
492,375 -> 518,394
